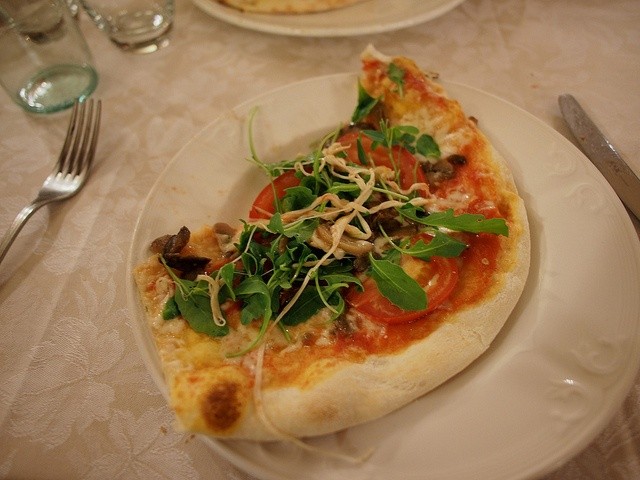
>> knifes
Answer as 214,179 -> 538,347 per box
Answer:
558,93 -> 640,216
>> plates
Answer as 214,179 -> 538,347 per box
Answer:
126,73 -> 639,480
194,1 -> 463,39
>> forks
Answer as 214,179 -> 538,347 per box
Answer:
1,98 -> 102,256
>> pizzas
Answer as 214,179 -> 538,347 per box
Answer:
131,44 -> 530,443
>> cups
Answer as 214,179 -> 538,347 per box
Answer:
82,0 -> 175,51
0,0 -> 99,114
